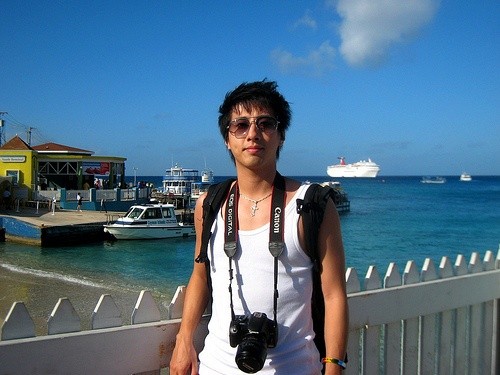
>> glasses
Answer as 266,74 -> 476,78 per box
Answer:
226,116 -> 280,139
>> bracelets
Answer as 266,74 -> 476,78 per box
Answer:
323,358 -> 346,368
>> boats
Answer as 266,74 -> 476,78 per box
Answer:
326,155 -> 380,178
458,171 -> 472,183
102,199 -> 196,239
420,175 -> 447,184
148,163 -> 219,212
319,181 -> 351,212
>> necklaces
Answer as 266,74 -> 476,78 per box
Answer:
240,194 -> 272,216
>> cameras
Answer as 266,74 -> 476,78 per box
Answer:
229,312 -> 279,374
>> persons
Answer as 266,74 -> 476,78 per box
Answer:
4,188 -> 11,201
162,188 -> 170,203
84,181 -> 90,190
77,193 -> 84,212
169,77 -> 347,375
95,181 -> 100,190
